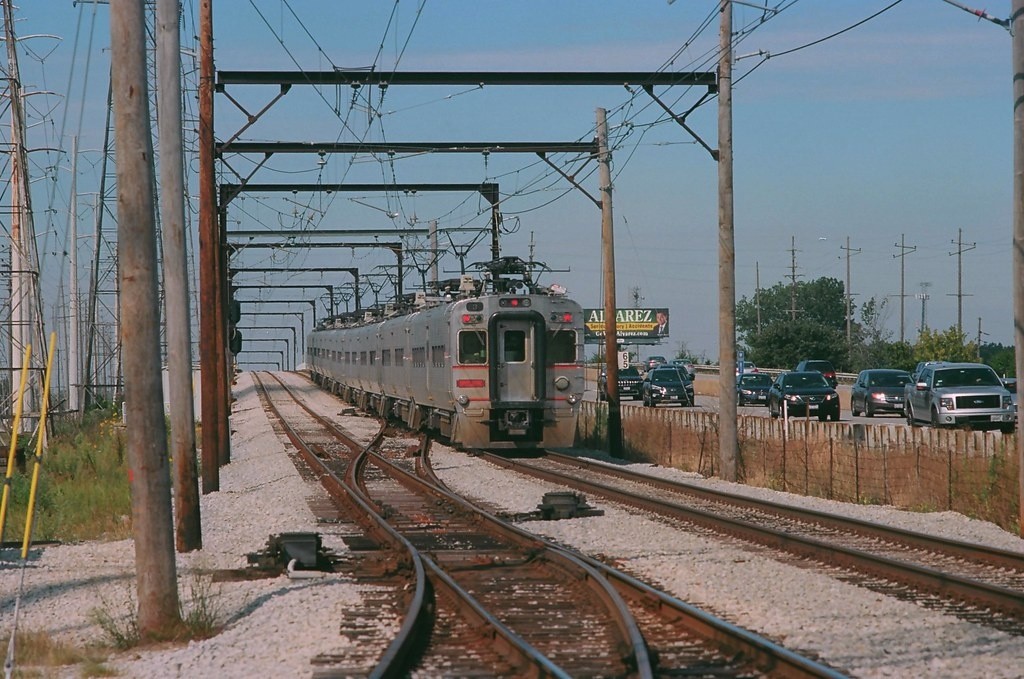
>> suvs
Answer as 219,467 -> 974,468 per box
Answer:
795,360 -> 838,390
597,364 -> 643,402
642,368 -> 696,408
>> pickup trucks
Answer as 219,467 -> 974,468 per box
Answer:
904,361 -> 1015,434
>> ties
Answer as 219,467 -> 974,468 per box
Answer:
660,327 -> 662,333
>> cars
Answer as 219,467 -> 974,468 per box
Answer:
656,363 -> 695,381
997,378 -> 1017,413
851,368 -> 914,417
767,369 -> 840,422
642,355 -> 668,372
670,359 -> 696,379
736,360 -> 759,377
735,373 -> 774,407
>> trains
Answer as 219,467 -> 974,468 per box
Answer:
306,229 -> 586,458
